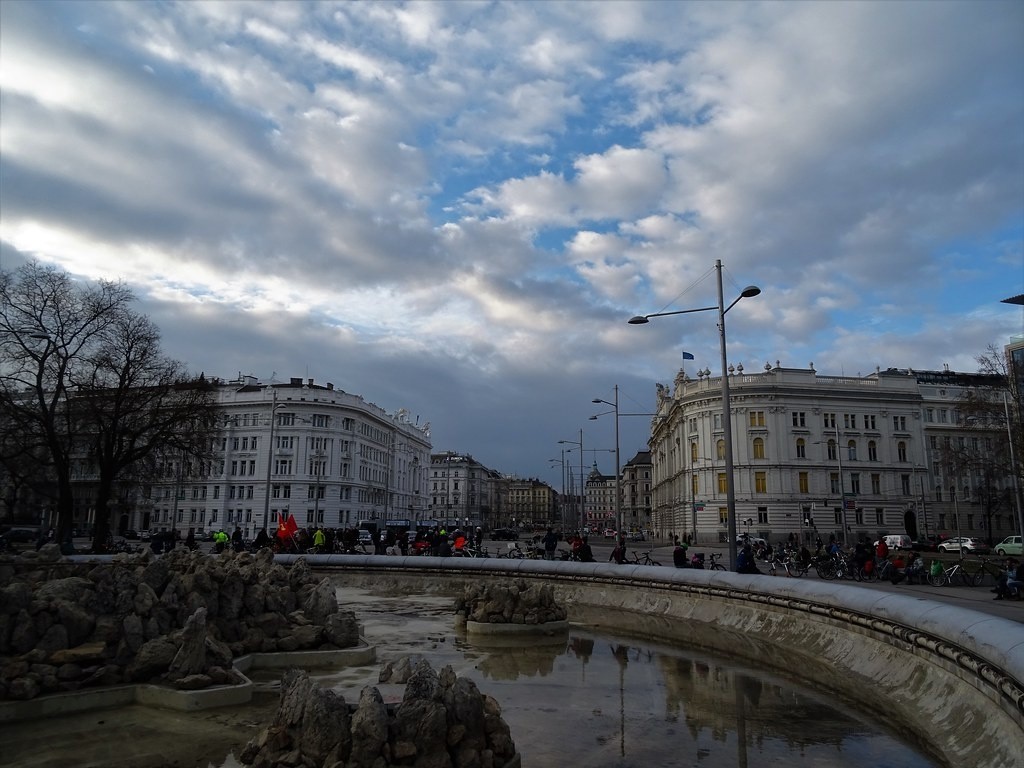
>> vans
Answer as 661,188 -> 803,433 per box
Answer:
994,536 -> 1023,556
874,535 -> 912,551
0,530 -> 34,543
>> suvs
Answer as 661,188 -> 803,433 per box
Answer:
357,530 -> 372,545
122,529 -> 153,539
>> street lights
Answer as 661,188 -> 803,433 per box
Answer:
354,434 -> 414,529
549,449 -> 591,541
588,384 -> 669,546
557,428 -> 616,539
812,440 -> 855,545
232,390 -> 312,535
627,259 -> 761,571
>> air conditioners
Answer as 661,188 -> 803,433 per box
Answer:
343,453 -> 350,459
316,450 -> 324,456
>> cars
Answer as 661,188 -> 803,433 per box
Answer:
194,532 -> 204,540
727,534 -> 766,546
937,537 -> 988,554
578,527 -> 652,541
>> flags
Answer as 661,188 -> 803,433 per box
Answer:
683,352 -> 694,359
277,515 -> 289,543
287,515 -> 297,531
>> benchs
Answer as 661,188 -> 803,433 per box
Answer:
896,570 -> 929,585
1007,580 -> 1024,601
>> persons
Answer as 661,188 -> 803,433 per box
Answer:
185,529 -> 194,545
669,532 -> 693,568
43,527 -> 55,539
253,527 -> 359,553
386,529 -> 409,555
745,532 -> 810,577
736,544 -> 763,574
855,536 -> 923,585
566,531 -> 596,561
816,531 -> 839,553
373,528 -> 381,554
415,526 -> 481,556
214,527 -> 242,553
150,528 -> 180,552
607,535 -> 626,563
990,557 -> 1024,600
542,527 -> 557,559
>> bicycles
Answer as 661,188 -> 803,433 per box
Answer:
926,555 -> 1006,587
704,552 -> 727,571
765,548 -> 894,583
452,535 -> 662,566
